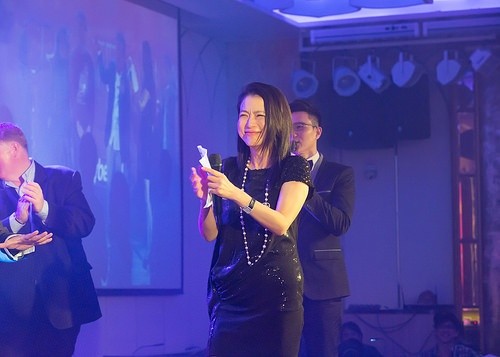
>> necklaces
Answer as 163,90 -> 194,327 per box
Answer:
239,156 -> 270,266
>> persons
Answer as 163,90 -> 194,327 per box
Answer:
341,321 -> 383,357
420,311 -> 482,357
189,83 -> 314,357
20,13 -> 178,208
0,227 -> 53,263
0,121 -> 102,357
289,98 -> 355,357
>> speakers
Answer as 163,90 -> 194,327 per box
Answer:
331,49 -> 430,149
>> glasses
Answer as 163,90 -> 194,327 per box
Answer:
293,124 -> 316,131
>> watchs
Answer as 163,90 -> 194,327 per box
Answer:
243,197 -> 255,214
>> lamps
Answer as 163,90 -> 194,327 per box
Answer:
422,16 -> 500,38
434,47 -> 464,86
358,53 -> 391,95
309,22 -> 421,47
290,50 -> 317,100
468,44 -> 498,80
328,51 -> 362,96
391,48 -> 426,88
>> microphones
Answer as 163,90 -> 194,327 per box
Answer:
291,141 -> 297,152
208,154 -> 223,227
